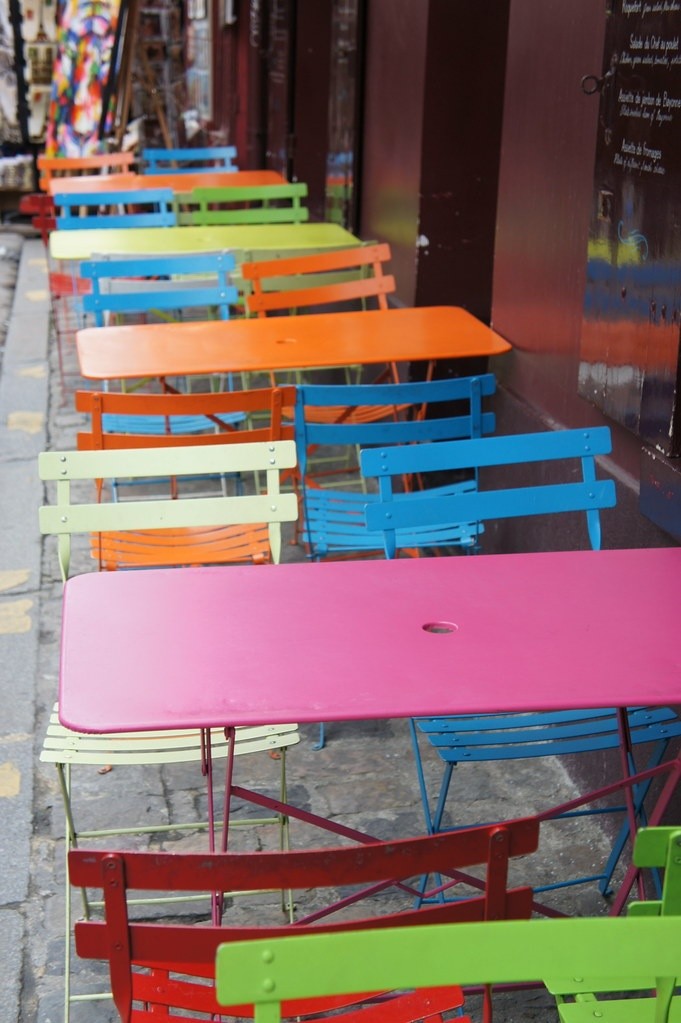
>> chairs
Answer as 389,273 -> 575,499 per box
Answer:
33,441 -> 300,1021
67,816 -> 540,1023
214,825 -> 681,1023
359,424 -> 680,915
22,144 -> 515,580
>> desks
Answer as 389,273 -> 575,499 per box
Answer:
62,545 -> 679,932
